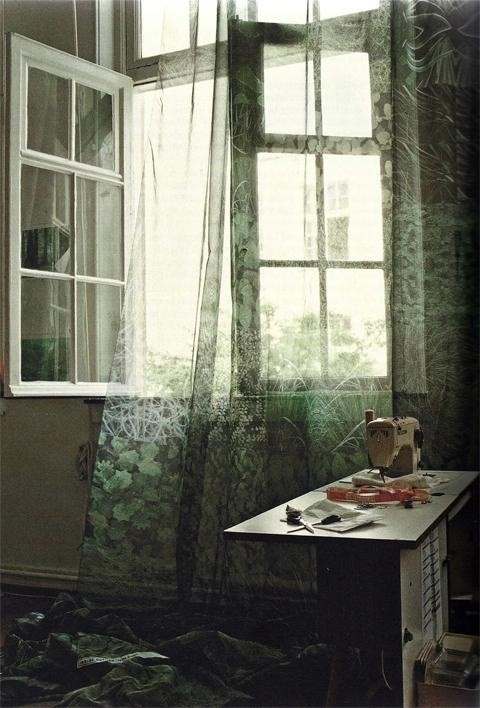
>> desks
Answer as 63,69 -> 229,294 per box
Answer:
221,467 -> 479,708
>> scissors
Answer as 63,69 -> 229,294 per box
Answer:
287,510 -> 341,534
